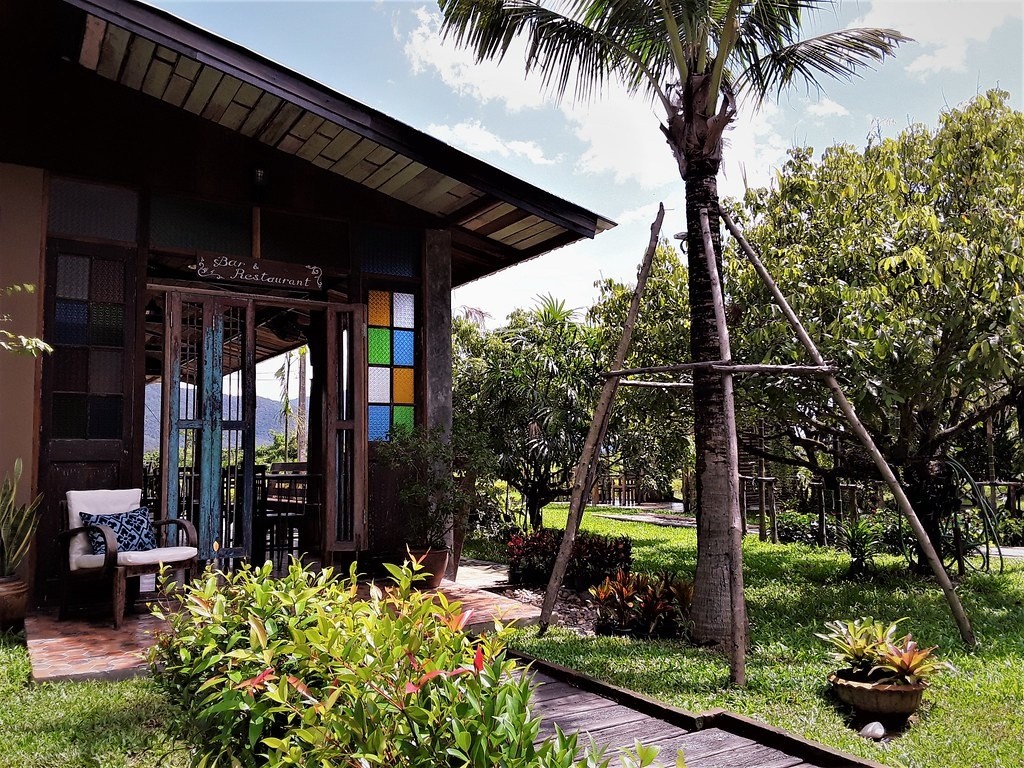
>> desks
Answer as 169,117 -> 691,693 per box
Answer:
266,473 -> 306,484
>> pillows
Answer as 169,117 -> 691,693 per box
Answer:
79,507 -> 158,556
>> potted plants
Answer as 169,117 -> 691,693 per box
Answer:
0,458 -> 43,635
818,615 -> 953,733
371,422 -> 500,589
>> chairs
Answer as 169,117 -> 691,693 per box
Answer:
64,488 -> 198,629
150,462 -> 313,573
